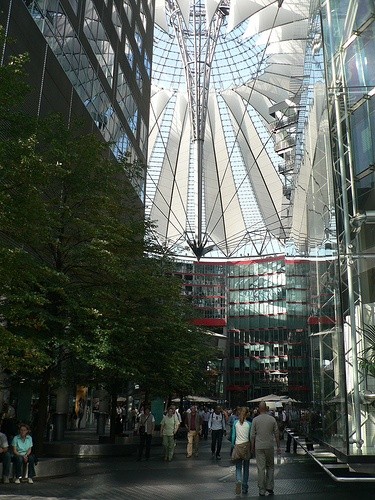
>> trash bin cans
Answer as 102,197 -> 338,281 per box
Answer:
48,423 -> 56,442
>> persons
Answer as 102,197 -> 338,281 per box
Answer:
184,406 -> 203,458
163,407 -> 177,461
250,403 -> 281,496
11,424 -> 35,484
0,431 -> 11,484
231,407 -> 252,495
117,403 -> 288,435
285,406 -> 298,454
208,405 -> 226,459
137,407 -> 154,462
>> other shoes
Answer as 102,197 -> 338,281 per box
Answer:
28,478 -> 33,484
15,479 -> 20,484
4,476 -> 9,483
235,480 -> 242,494
259,489 -> 274,496
218,456 -> 222,460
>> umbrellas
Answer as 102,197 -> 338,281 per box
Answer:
171,396 -> 216,407
247,394 -> 302,403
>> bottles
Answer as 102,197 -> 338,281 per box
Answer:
277,453 -> 281,465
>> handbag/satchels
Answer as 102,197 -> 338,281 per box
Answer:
231,442 -> 252,460
